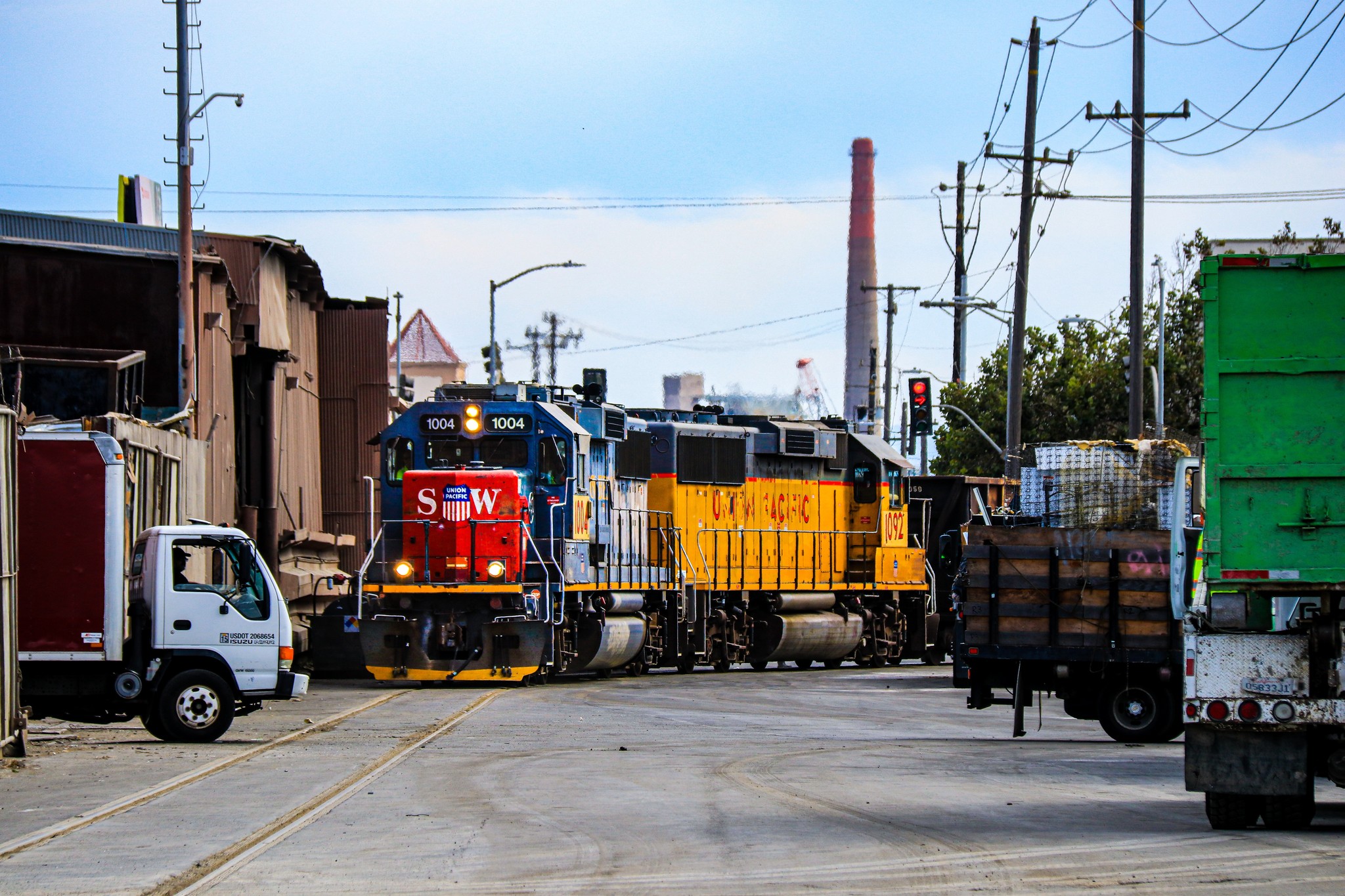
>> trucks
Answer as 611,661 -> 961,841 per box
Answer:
17,430 -> 311,743
949,456 -> 1206,744
1183,251 -> 1345,836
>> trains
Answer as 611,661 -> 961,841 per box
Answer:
331,378 -> 937,684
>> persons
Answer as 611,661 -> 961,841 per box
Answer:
172,547 -> 233,595
397,450 -> 412,480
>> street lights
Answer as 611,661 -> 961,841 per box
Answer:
489,260 -> 588,388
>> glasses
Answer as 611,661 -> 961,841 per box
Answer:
180,558 -> 188,562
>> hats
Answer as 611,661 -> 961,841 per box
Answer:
173,547 -> 191,558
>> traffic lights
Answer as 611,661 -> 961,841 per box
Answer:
1121,356 -> 1131,394
908,377 -> 933,436
